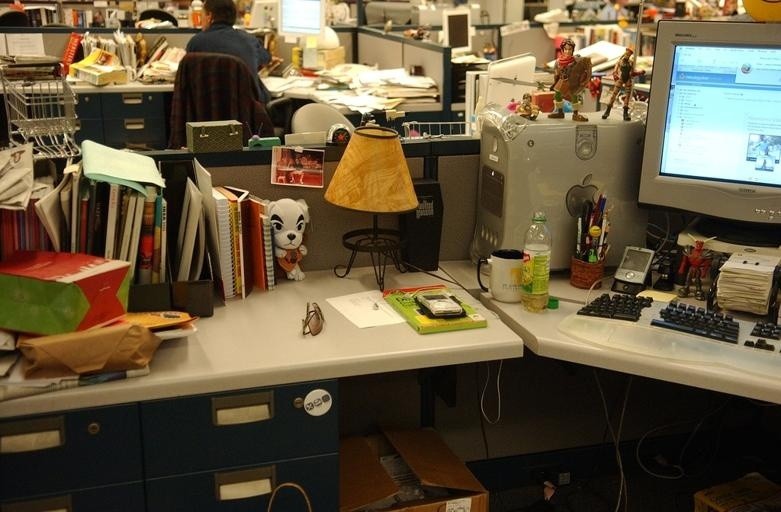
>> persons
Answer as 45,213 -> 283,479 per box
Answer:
602,48 -> 646,121
185,1 -> 273,105
548,39 -> 589,122
516,93 -> 541,120
278,149 -> 322,184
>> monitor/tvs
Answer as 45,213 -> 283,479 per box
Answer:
637,19 -> 781,254
248,0 -> 278,30
443,9 -> 471,58
278,0 -> 324,48
484,53 -> 538,135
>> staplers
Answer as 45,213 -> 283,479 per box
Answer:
653,260 -> 674,291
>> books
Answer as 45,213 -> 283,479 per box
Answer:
1,159 -> 275,313
249,29 -> 440,110
8,33 -> 187,87
546,23 -> 654,85
19,2 -> 281,28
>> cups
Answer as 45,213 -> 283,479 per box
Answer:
478,248 -> 522,302
410,65 -> 422,76
570,254 -> 604,289
304,48 -> 327,71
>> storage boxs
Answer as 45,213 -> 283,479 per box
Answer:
340,427 -> 489,511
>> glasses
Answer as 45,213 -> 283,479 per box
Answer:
301,302 -> 324,336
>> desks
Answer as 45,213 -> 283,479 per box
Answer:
3,255 -> 526,510
449,253 -> 781,511
0,49 -> 443,143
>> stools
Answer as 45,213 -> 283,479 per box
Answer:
694,472 -> 781,511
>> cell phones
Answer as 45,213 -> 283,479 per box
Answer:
417,294 -> 463,317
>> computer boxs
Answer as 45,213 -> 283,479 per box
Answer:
469,103 -> 652,274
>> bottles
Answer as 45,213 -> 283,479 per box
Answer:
520,212 -> 553,312
292,47 -> 303,70
192,0 -> 205,29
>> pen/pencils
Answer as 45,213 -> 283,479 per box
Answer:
576,192 -> 611,264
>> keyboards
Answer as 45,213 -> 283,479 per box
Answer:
558,291 -> 781,380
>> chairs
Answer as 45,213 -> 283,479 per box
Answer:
170,53 -> 273,150
